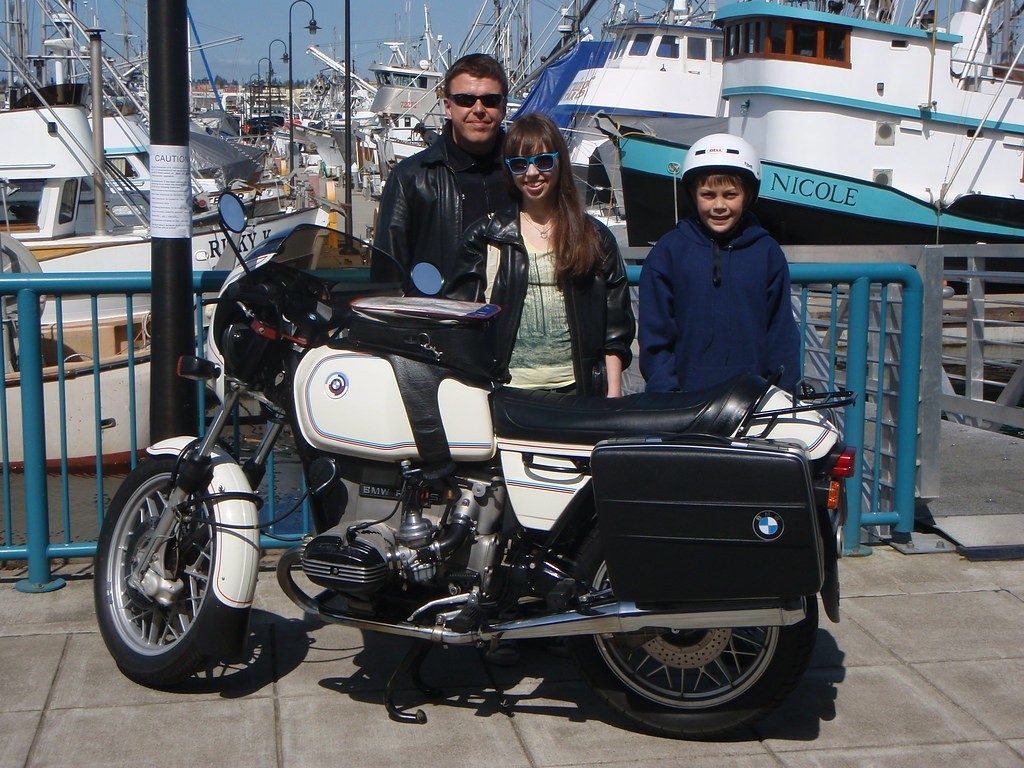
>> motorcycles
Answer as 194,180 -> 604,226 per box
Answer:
93,191 -> 858,738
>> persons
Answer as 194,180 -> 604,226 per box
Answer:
443,115 -> 637,397
376,54 -> 516,303
637,133 -> 799,395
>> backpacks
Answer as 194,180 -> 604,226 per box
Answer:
348,297 -> 512,490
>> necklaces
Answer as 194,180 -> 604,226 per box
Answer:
521,212 -> 553,238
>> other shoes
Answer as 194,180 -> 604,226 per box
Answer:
488,640 -> 519,665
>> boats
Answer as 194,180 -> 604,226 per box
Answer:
221,0 -> 1024,295
0,0 -> 326,474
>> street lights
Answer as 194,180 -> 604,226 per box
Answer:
269,39 -> 289,151
258,58 -> 276,135
287,0 -> 322,200
249,73 -> 265,134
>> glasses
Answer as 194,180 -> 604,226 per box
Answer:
446,93 -> 504,107
505,152 -> 559,174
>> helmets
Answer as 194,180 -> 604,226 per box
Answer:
681,134 -> 761,209
